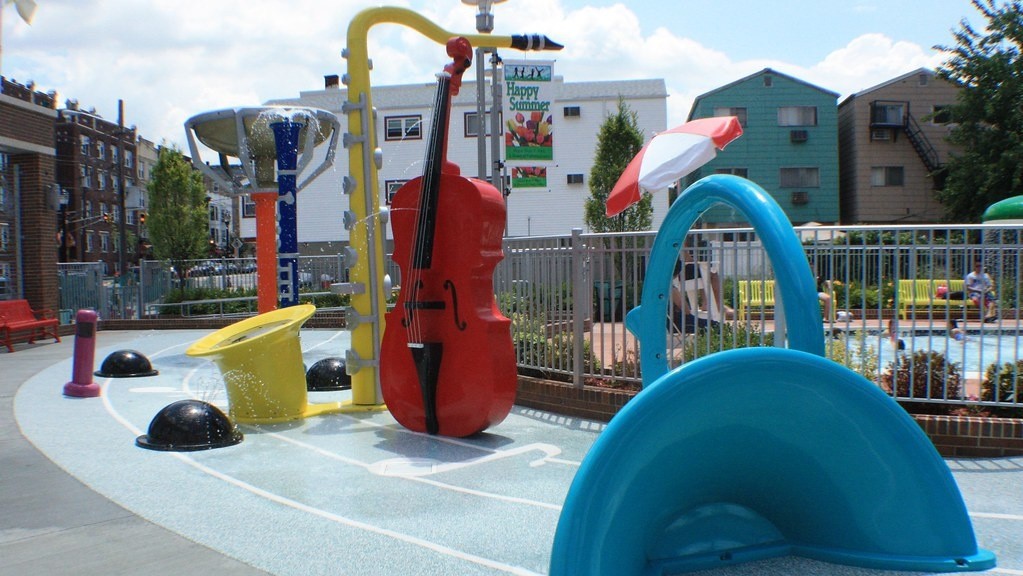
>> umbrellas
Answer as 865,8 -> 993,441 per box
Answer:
605,115 -> 743,219
795,222 -> 847,243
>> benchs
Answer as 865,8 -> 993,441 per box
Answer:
899,279 -> 996,321
0,299 -> 61,353
739,281 -> 837,322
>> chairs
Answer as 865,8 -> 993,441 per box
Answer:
667,314 -> 699,362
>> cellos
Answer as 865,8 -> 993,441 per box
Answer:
376,33 -> 521,440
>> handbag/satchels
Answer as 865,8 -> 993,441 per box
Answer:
935,286 -> 947,297
943,291 -> 968,300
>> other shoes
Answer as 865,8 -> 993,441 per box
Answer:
984,314 -> 998,323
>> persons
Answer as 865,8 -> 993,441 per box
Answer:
882,319 -> 895,334
950,319 -> 963,339
894,338 -> 905,348
667,259 -> 729,335
679,243 -> 735,312
966,261 -> 997,323
833,328 -> 845,342
810,263 -> 835,324
